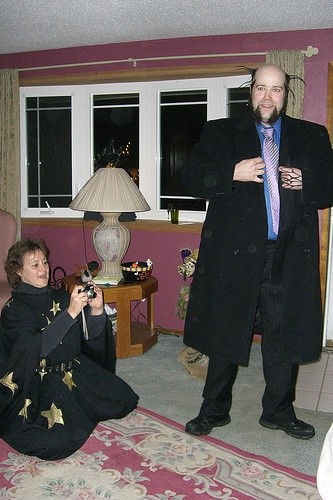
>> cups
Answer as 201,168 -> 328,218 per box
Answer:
107,314 -> 118,336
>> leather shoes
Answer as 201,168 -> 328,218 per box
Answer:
185,414 -> 231,435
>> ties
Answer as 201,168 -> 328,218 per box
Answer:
260,127 -> 280,235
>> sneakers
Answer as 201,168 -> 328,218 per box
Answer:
259,414 -> 314,440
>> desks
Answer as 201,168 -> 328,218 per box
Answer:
62,275 -> 159,360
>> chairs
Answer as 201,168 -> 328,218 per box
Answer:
1,209 -> 19,311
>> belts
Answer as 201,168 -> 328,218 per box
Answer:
38,353 -> 79,373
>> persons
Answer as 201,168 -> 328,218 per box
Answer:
0,239 -> 139,460
181,64 -> 333,440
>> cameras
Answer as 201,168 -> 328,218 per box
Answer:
78,284 -> 97,299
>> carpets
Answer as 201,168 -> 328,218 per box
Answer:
0,403 -> 322,500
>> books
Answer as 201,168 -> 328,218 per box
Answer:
91,276 -> 121,285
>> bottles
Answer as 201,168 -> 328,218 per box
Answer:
170,203 -> 178,224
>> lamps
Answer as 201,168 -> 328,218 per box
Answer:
68,167 -> 150,284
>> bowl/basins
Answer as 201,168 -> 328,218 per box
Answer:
121,262 -> 150,282
